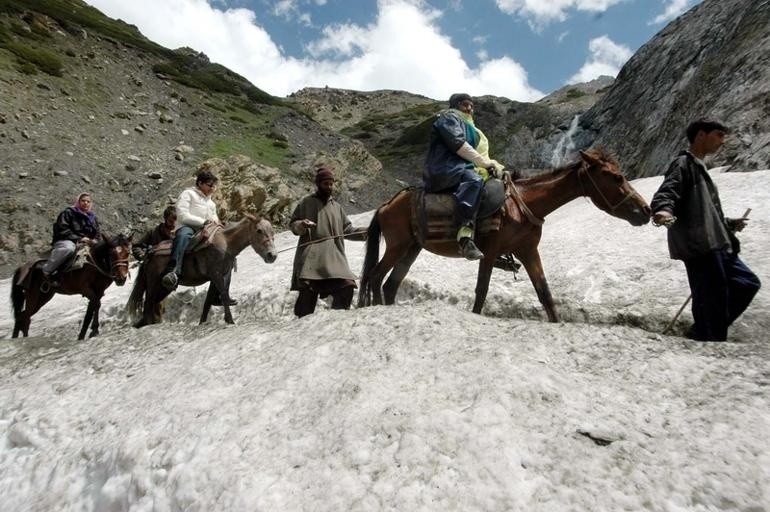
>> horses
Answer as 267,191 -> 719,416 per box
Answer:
353,147 -> 652,323
125,208 -> 277,328
10,230 -> 137,342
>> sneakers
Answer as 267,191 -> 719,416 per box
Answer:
457,238 -> 485,260
493,255 -> 521,271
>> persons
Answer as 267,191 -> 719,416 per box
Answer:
40,191 -> 101,282
421,92 -> 508,261
289,168 -> 372,318
131,204 -> 178,329
650,116 -> 762,342
160,166 -> 239,307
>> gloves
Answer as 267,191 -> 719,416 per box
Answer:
457,141 -> 505,172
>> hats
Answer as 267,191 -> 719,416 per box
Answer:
449,93 -> 475,106
315,168 -> 334,186
